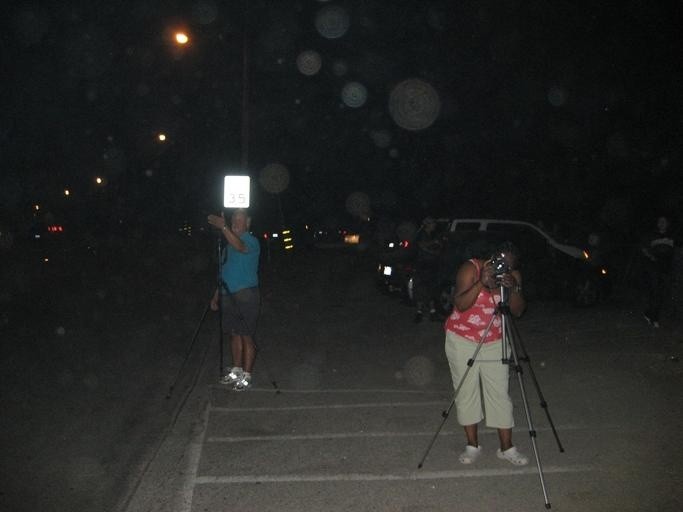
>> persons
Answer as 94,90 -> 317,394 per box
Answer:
411,215 -> 445,325
440,238 -> 530,468
205,207 -> 261,392
637,214 -> 675,331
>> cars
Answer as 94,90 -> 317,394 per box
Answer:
366,218 -> 612,321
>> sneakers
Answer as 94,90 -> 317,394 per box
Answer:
218,365 -> 253,391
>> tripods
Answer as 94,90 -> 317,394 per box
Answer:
165,208 -> 281,401
417,279 -> 565,509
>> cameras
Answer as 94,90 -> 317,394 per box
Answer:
492,255 -> 507,280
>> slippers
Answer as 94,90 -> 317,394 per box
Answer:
458,442 -> 483,465
495,445 -> 530,468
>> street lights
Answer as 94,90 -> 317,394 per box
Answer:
174,30 -> 250,175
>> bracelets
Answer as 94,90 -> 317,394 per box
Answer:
478,279 -> 485,287
222,224 -> 227,232
